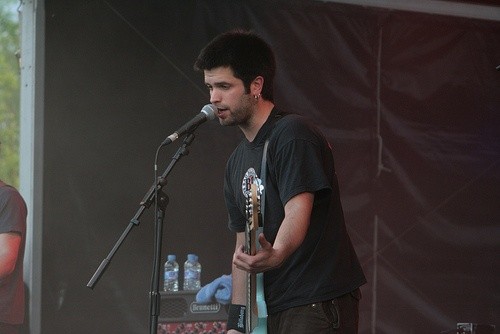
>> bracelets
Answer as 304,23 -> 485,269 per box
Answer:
225,304 -> 246,332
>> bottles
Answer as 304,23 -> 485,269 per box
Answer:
163,255 -> 179,291
182,254 -> 202,291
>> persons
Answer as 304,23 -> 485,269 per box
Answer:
0,182 -> 27,334
193,28 -> 365,334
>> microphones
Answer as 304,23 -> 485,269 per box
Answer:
159,104 -> 218,146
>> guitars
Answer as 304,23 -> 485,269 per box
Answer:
241,167 -> 265,334
